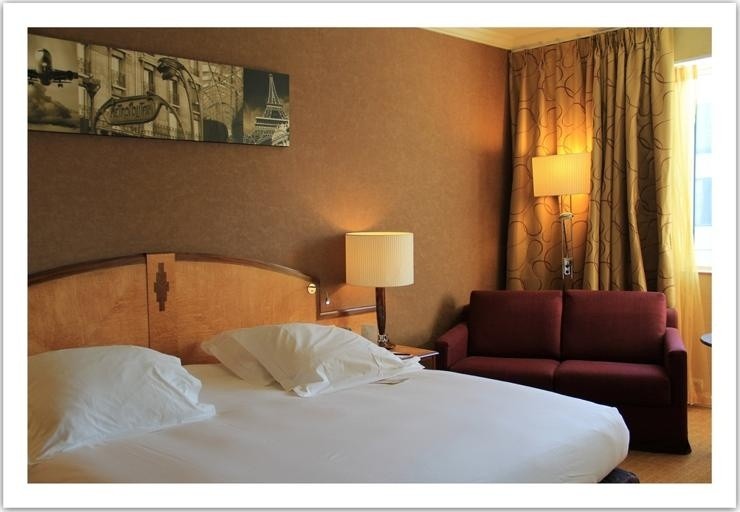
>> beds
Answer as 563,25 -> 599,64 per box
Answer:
27,251 -> 631,483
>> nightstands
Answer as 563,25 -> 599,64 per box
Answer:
387,344 -> 440,369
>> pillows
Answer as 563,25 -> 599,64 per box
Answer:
26,343 -> 215,467
468,289 -> 564,358
563,290 -> 666,365
201,322 -> 425,396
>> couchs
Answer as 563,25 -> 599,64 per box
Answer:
432,308 -> 691,456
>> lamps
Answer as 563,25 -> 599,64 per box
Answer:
532,152 -> 592,288
346,231 -> 414,348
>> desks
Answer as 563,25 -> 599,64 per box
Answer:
700,333 -> 712,348
